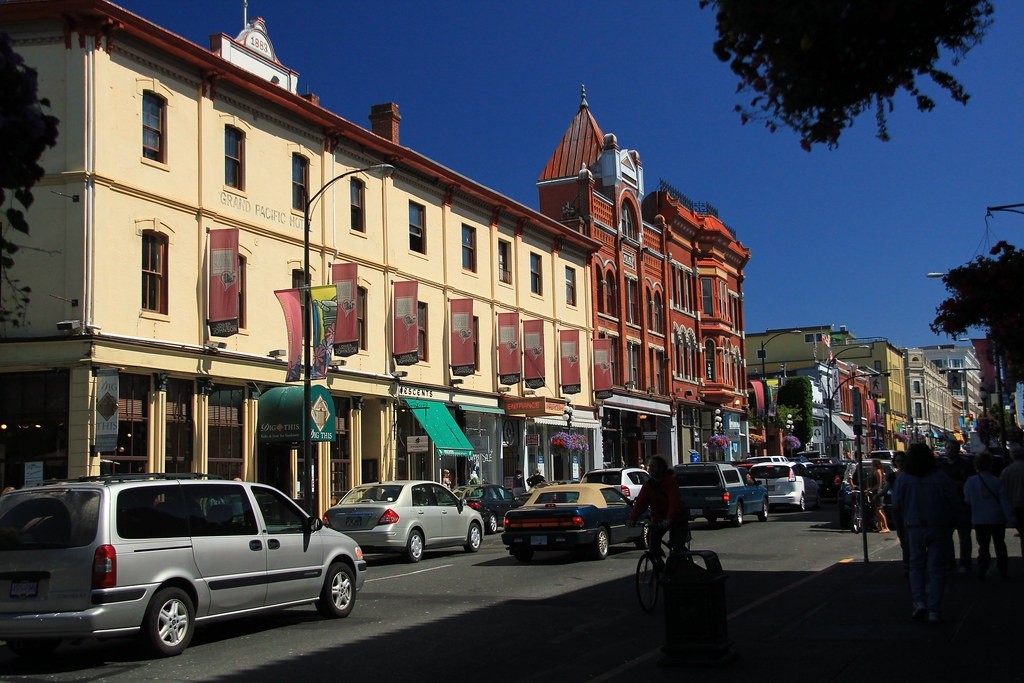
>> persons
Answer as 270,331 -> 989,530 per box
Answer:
882,442 -> 952,619
443,469 -> 451,490
526,468 -> 546,488
513,469 -> 523,495
963,452 -> 1013,580
867,459 -> 891,533
872,378 -> 880,390
840,450 -> 865,529
1000,446 -> 1024,557
621,455 -> 689,585
950,374 -> 959,387
940,440 -> 975,573
814,429 -> 821,442
645,455 -> 651,465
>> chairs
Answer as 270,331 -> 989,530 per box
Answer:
381,489 -> 429,507
186,499 -> 237,536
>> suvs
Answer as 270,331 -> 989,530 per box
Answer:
579,467 -> 651,500
673,461 -> 769,527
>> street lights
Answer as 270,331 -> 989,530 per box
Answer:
714,409 -> 724,460
758,329 -> 802,455
926,272 -> 1007,456
959,338 -> 991,450
563,395 -> 576,481
827,345 -> 871,455
303,164 -> 396,518
786,414 -> 795,455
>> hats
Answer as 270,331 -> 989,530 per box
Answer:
515,470 -> 522,474
650,456 -> 667,473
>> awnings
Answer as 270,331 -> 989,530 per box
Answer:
258,384 -> 336,442
931,427 -> 942,438
405,399 -> 473,456
825,414 -> 855,439
945,433 -> 956,441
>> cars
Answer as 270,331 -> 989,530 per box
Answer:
841,461 -> 897,532
322,480 -> 485,562
452,484 -> 524,535
502,482 -> 649,563
745,455 -> 858,512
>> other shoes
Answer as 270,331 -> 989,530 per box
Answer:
912,608 -> 928,623
929,612 -> 944,623
958,567 -> 969,573
904,571 -> 909,579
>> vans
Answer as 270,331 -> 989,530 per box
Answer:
0,473 -> 368,656
870,451 -> 897,460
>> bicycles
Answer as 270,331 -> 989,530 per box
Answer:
845,486 -> 872,534
624,519 -> 691,613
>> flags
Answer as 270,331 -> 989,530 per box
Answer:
823,333 -> 830,347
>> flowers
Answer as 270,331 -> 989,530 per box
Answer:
854,436 -> 866,445
894,432 -> 925,444
550,431 -> 590,455
781,435 -> 802,451
749,433 -> 764,446
706,434 -> 731,452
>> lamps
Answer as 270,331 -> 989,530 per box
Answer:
497,387 -> 511,394
207,340 -> 228,350
329,360 -> 346,368
392,371 -> 408,381
624,381 -> 694,399
522,390 -> 536,396
269,350 -> 287,357
449,379 -> 463,388
56,320 -> 80,330
